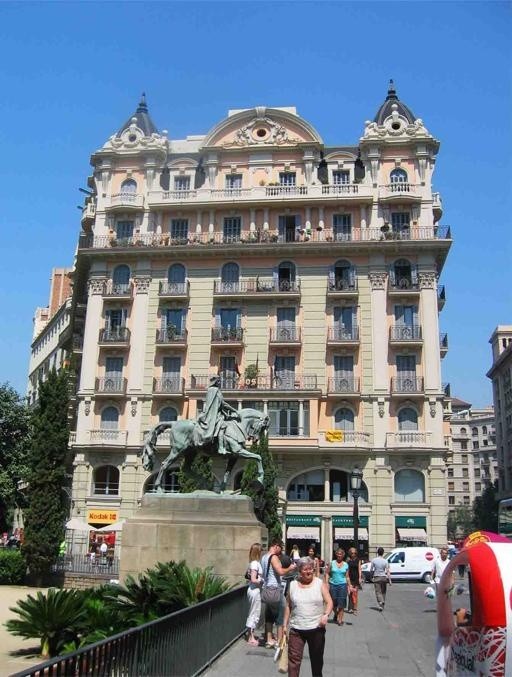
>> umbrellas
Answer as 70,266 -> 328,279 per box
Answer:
247,637 -> 261,645
333,614 -> 342,627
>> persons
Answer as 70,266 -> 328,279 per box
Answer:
368,547 -> 392,612
90,530 -> 114,558
205,375 -> 241,455
458,565 -> 466,579
323,547 -> 363,625
289,544 -> 301,563
245,538 -> 297,646
381,221 -> 392,240
396,555 -> 402,563
430,549 -> 456,595
306,545 -> 320,577
455,608 -> 472,627
282,557 -> 334,677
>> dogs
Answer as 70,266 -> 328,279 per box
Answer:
140,406 -> 272,494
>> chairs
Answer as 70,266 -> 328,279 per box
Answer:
264,640 -> 281,648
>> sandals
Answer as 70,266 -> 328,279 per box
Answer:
497,498 -> 512,538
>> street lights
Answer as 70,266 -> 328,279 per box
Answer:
423,587 -> 436,600
245,569 -> 258,579
262,587 -> 282,604
274,643 -> 291,673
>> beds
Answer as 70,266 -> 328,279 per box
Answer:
94,519 -> 128,532
63,518 -> 97,555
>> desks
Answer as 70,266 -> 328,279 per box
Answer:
349,463 -> 363,549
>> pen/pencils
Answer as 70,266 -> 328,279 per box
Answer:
361,547 -> 440,583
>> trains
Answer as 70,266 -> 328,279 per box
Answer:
425,552 -> 434,560
